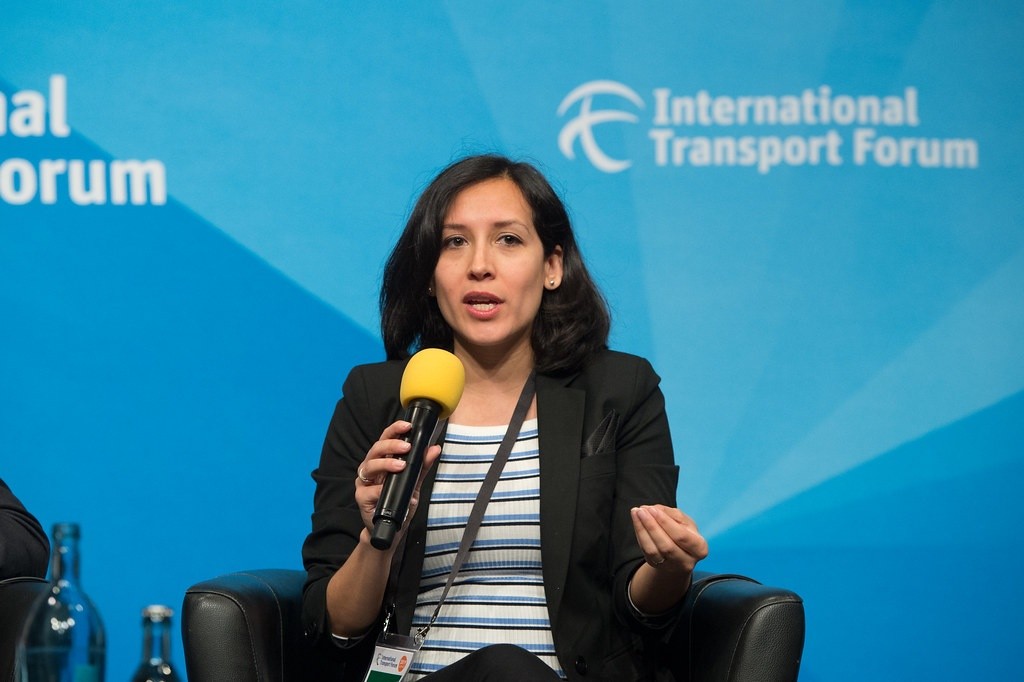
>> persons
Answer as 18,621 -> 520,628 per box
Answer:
0,477 -> 50,581
296,154 -> 707,682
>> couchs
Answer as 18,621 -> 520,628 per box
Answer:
181,570 -> 806,682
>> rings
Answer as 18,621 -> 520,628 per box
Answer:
657,558 -> 665,564
359,467 -> 375,482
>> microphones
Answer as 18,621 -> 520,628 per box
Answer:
369,347 -> 467,551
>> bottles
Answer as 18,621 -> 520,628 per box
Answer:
129,605 -> 182,682
13,523 -> 107,682
